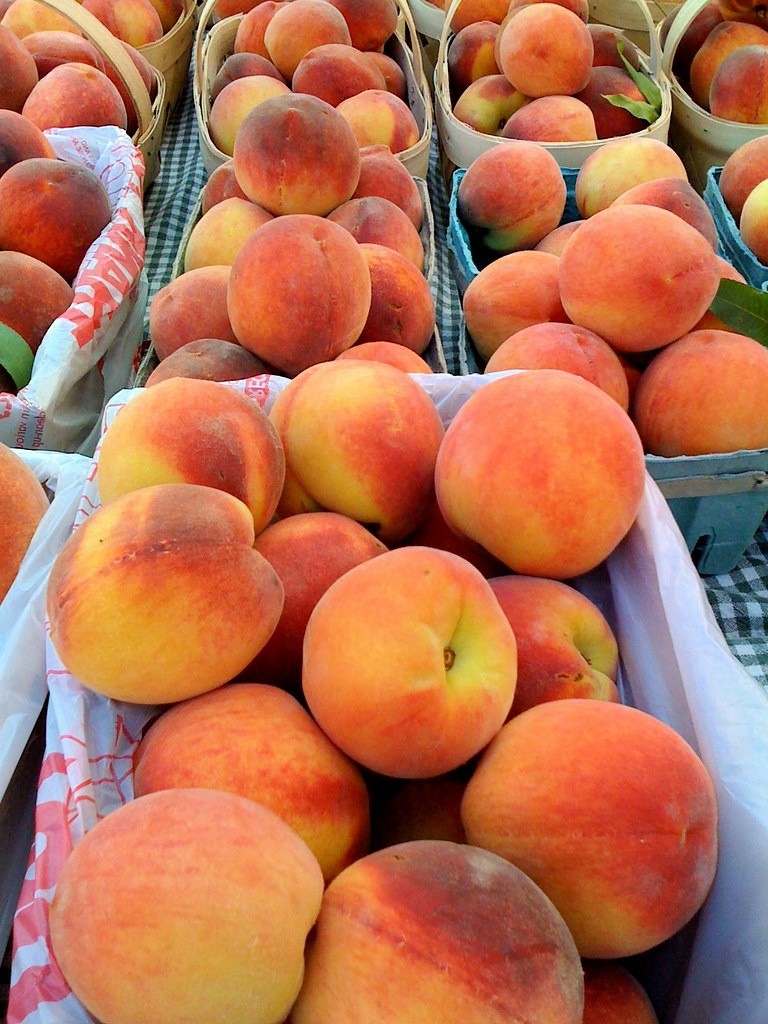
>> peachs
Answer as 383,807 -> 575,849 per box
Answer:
0,0 -> 768,1024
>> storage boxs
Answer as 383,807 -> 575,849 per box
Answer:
140,162 -> 767,683
